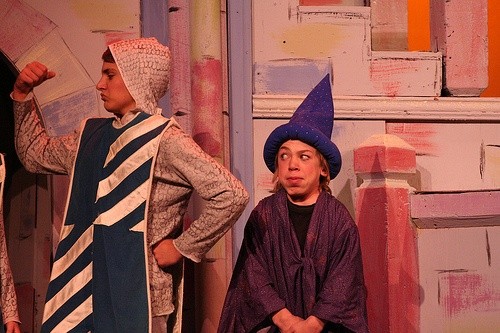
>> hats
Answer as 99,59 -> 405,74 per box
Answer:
264,72 -> 342,180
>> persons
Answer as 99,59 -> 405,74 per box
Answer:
216,72 -> 369,333
9,36 -> 250,333
0,153 -> 22,333
